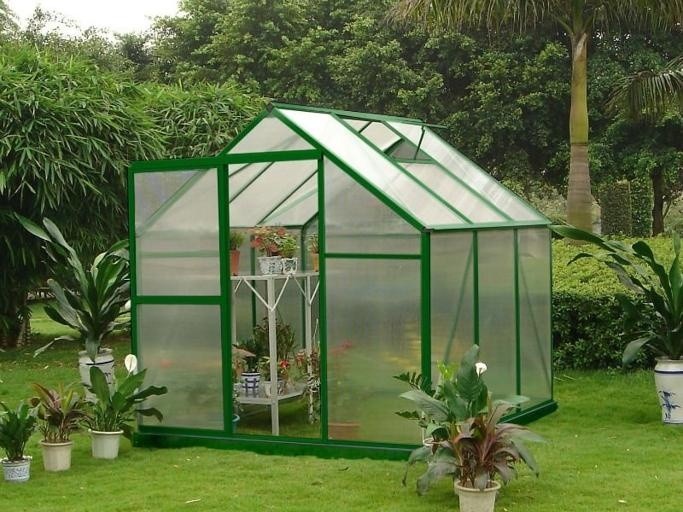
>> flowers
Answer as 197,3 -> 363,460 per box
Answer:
326,337 -> 359,424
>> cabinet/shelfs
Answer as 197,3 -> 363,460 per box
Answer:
231,271 -> 319,439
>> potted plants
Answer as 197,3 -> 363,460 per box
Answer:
0,399 -> 42,484
547,215 -> 683,427
226,223 -> 319,274
78,354 -> 167,462
393,342 -> 548,512
231,396 -> 245,435
232,313 -> 318,398
26,379 -> 94,473
14,212 -> 134,400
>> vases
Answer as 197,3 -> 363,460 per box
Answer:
329,421 -> 359,440
